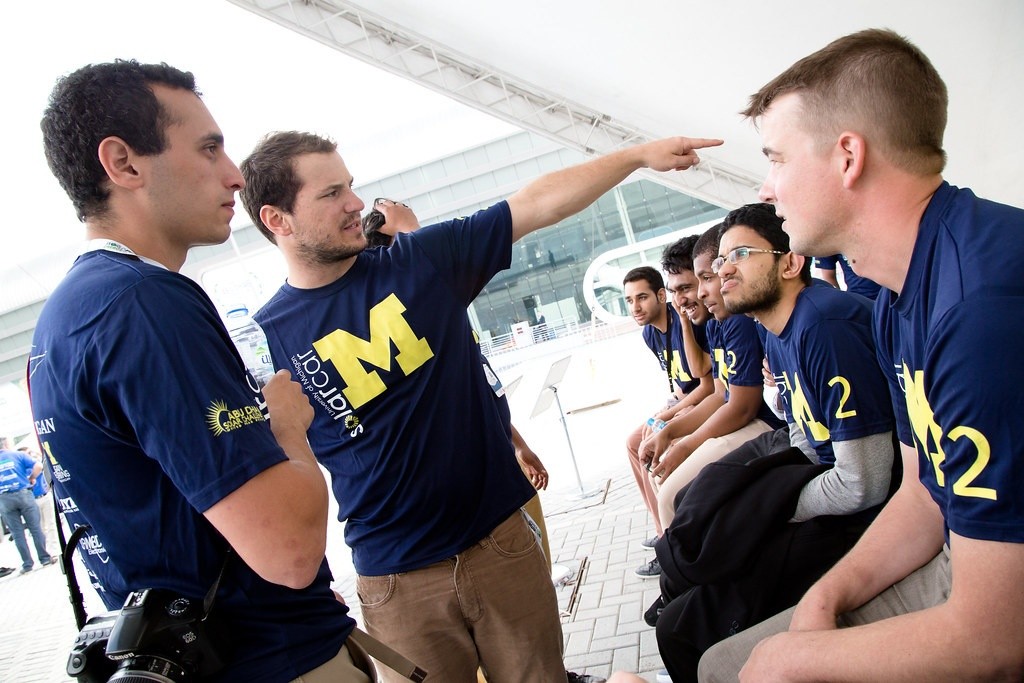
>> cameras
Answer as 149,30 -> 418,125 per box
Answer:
67,586 -> 222,683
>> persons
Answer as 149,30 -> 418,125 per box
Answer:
810,251 -> 899,306
620,222 -> 788,578
0,438 -> 65,577
696,31 -> 1023,683
358,197 -> 606,682
27,55 -> 430,683
656,204 -> 904,683
236,129 -> 725,683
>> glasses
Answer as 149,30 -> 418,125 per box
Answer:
711,247 -> 787,273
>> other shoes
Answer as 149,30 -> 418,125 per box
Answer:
20,565 -> 33,574
644,594 -> 666,626
42,556 -> 58,567
566,671 -> 606,683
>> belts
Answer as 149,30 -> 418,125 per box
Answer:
349,627 -> 427,683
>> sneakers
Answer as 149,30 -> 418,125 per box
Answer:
635,557 -> 661,578
641,535 -> 658,549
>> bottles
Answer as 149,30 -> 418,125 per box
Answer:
647,418 -> 667,430
225,304 -> 275,389
